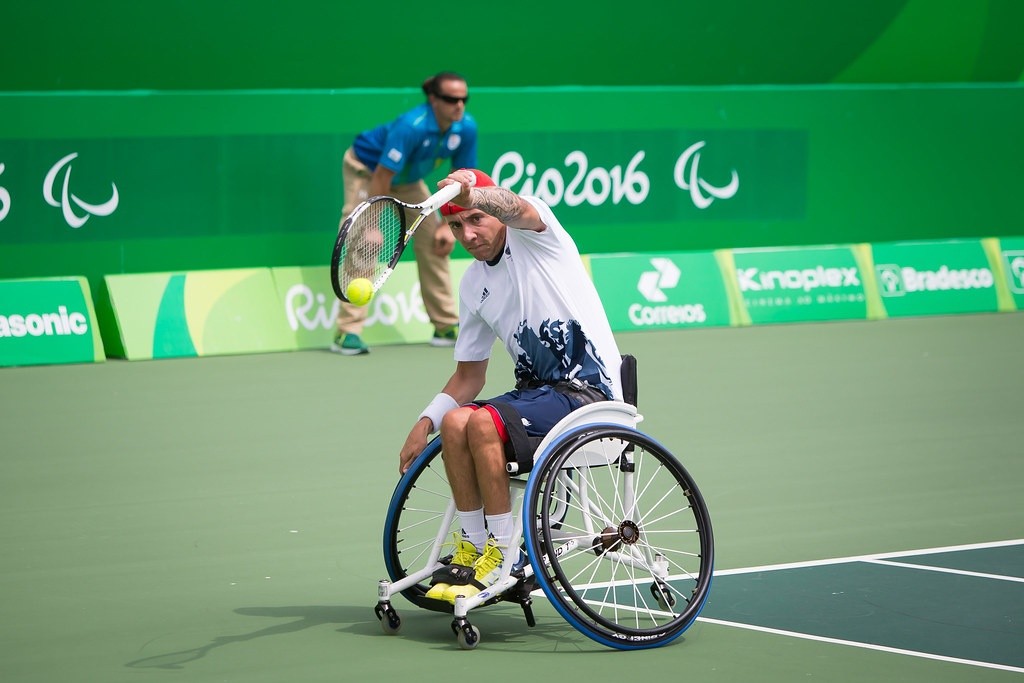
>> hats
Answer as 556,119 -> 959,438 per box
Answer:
441,169 -> 497,216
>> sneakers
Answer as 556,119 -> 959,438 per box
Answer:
425,528 -> 488,599
441,537 -> 530,605
330,328 -> 370,355
431,321 -> 460,346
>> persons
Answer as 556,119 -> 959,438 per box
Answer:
398,166 -> 627,605
328,71 -> 481,357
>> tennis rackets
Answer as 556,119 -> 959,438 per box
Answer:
328,170 -> 479,305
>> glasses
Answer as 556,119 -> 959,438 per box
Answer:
435,90 -> 470,105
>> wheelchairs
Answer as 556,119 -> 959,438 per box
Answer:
374,352 -> 715,650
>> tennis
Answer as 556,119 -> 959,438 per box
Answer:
345,278 -> 374,307
